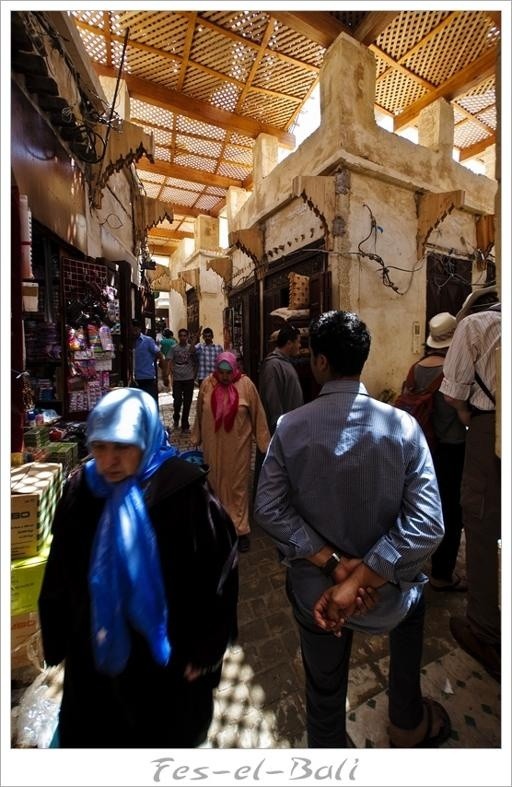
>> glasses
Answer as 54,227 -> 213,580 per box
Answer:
291,325 -> 295,331
204,335 -> 211,337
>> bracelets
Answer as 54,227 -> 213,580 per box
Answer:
324,553 -> 341,574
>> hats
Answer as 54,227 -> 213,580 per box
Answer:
456,284 -> 499,323
425,312 -> 458,350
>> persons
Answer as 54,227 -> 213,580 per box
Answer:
394,313 -> 472,593
132,321 -> 304,551
253,308 -> 446,748
37,386 -> 237,748
439,284 -> 501,684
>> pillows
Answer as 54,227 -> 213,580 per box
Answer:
287,273 -> 309,308
271,306 -> 310,356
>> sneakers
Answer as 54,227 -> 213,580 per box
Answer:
237,535 -> 251,553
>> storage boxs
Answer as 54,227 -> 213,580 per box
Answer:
11,607 -> 46,686
11,460 -> 63,559
11,534 -> 55,612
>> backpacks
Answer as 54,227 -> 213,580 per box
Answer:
393,356 -> 445,455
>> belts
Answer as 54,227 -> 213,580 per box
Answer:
470,410 -> 498,418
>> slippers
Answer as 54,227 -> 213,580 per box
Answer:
387,698 -> 452,748
430,572 -> 470,592
